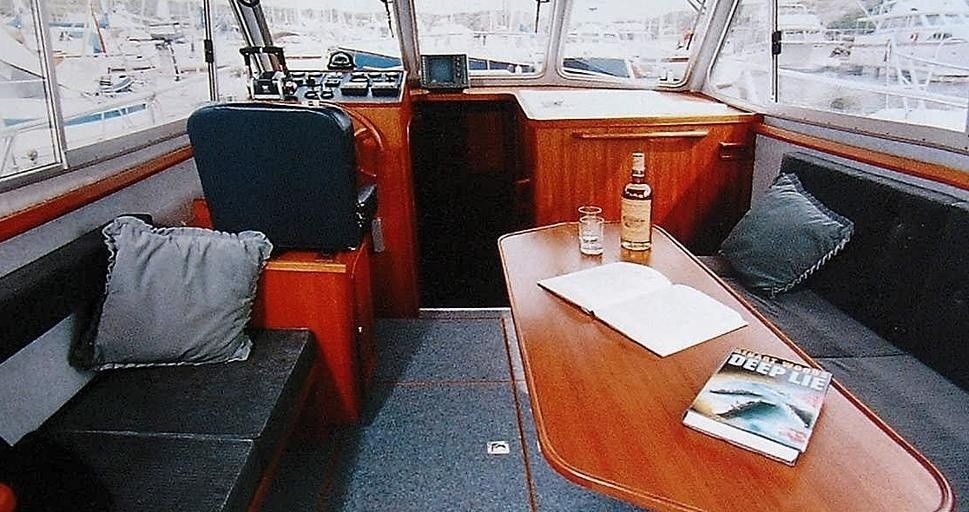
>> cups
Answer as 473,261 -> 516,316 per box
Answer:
577,206 -> 605,255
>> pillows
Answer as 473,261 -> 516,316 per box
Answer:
718,171 -> 854,299
92,214 -> 275,373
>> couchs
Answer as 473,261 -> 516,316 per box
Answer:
692,150 -> 968,511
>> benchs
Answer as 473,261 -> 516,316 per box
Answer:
0,213 -> 322,511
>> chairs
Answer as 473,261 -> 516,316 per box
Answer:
185,102 -> 378,254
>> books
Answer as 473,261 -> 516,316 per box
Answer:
534,258 -> 750,359
687,345 -> 835,453
681,409 -> 800,467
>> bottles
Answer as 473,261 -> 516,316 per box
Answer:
620,152 -> 654,264
